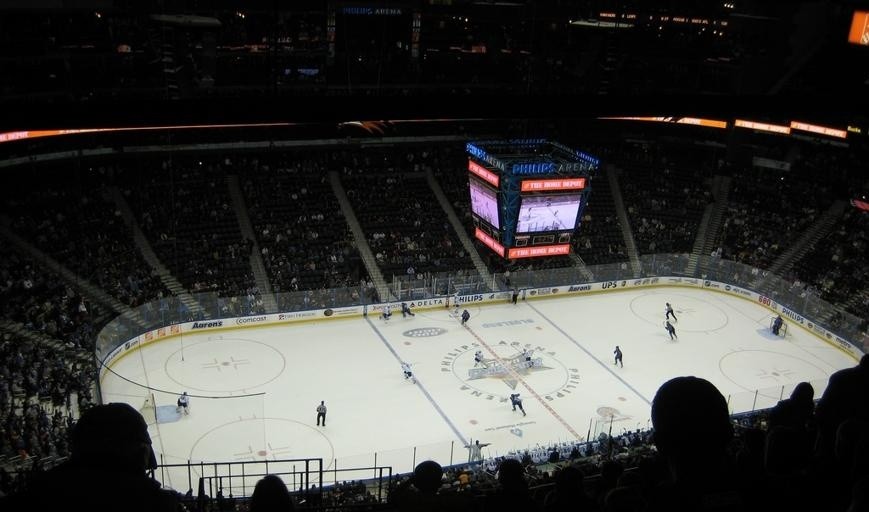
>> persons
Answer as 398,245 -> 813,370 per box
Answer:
316,401 -> 327,426
461,310 -> 470,325
474,351 -> 484,366
1,153 -> 191,512
522,349 -> 534,367
401,362 -> 416,384
186,429 -> 651,512
613,346 -> 623,367
189,154 -> 652,323
652,140 -> 869,511
509,393 -> 527,416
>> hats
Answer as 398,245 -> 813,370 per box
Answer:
67,402 -> 158,470
415,461 -> 444,480
652,377 -> 730,424
